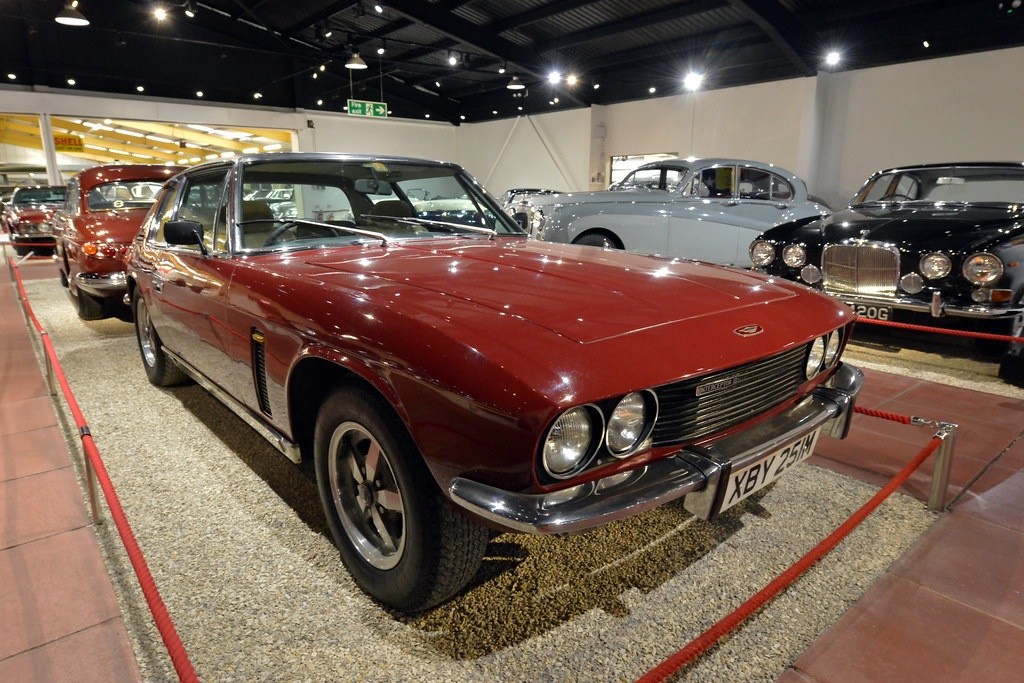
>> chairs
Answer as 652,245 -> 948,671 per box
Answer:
105,185 -> 135,202
219,198 -> 296,251
357,200 -> 430,235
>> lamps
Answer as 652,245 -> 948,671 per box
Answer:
507,75 -> 525,89
497,65 -> 505,74
54,0 -> 91,27
345,46 -> 368,70
185,2 -> 198,18
321,27 -> 332,38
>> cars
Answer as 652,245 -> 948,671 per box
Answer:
749,162 -> 1024,388
52,165 -> 188,321
504,158 -> 832,267
121,154 -> 865,609
2,185 -> 67,259
